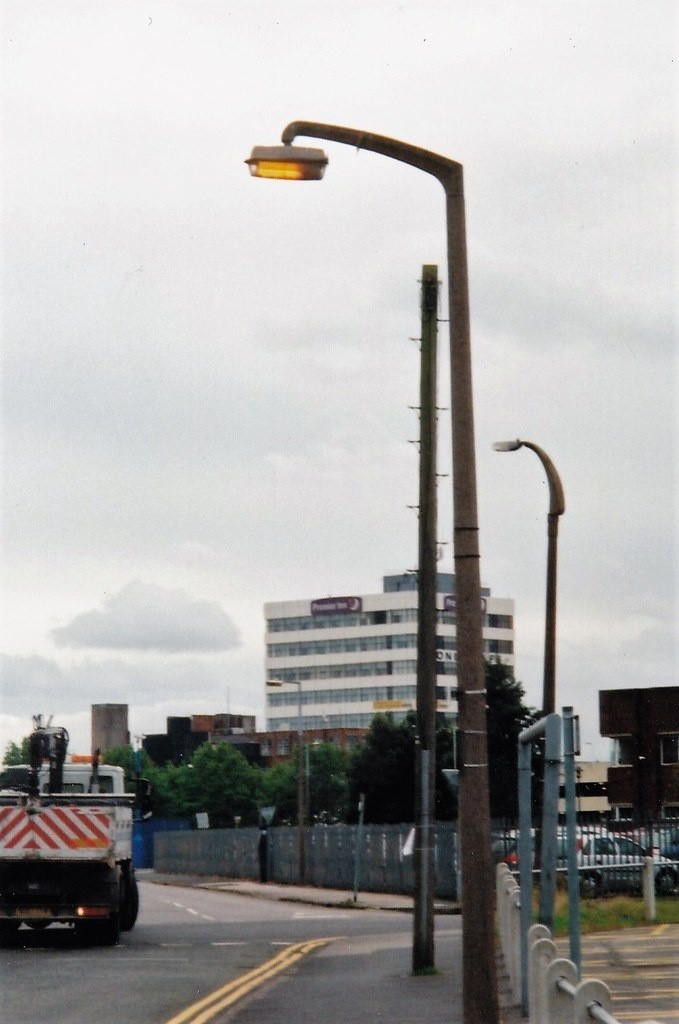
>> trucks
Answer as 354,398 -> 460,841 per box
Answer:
1,712 -> 139,952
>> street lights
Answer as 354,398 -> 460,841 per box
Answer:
264,678 -> 304,827
245,123 -> 502,1023
492,439 -> 565,871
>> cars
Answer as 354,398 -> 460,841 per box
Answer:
283,822 -> 677,899
